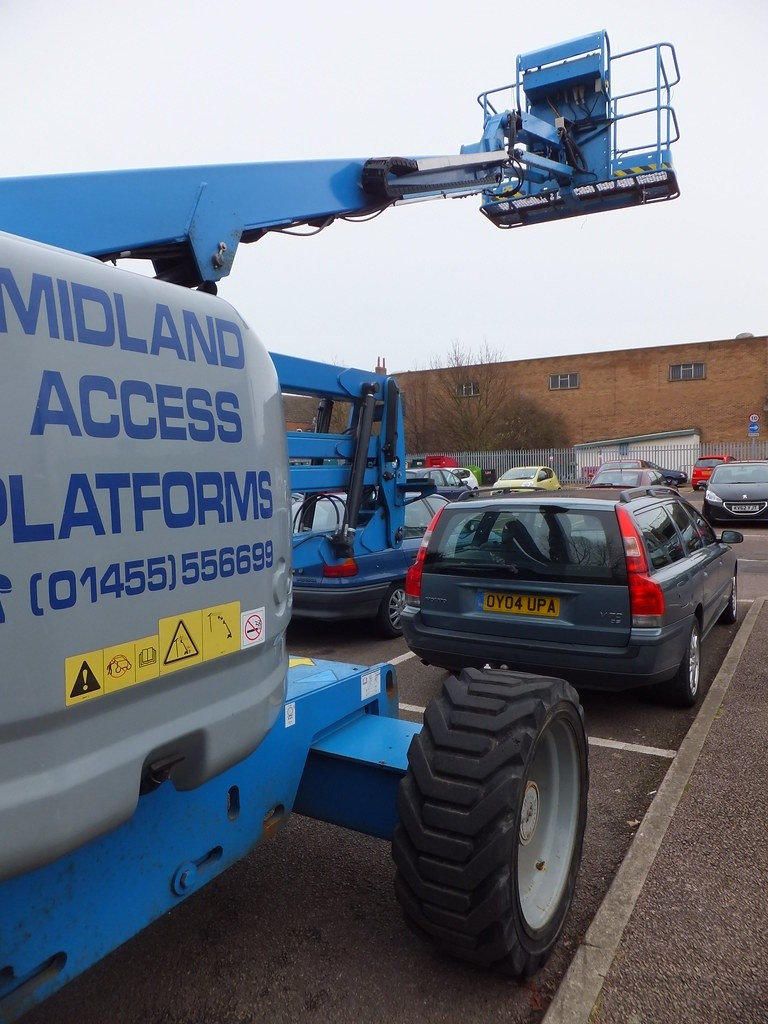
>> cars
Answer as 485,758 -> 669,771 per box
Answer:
285,466 -> 481,640
700,460 -> 768,528
690,454 -> 737,491
583,460 -> 688,493
490,464 -> 563,497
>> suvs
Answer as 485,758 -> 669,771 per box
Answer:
397,483 -> 745,709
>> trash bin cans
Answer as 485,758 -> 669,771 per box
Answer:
410,459 -> 425,468
482,469 -> 495,485
425,455 -> 458,468
463,465 -> 482,486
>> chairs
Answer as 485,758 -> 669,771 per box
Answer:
718,469 -> 732,481
570,525 -> 606,567
501,521 -> 536,544
752,468 -> 768,480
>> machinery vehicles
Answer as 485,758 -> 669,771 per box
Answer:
0,27 -> 681,1024
